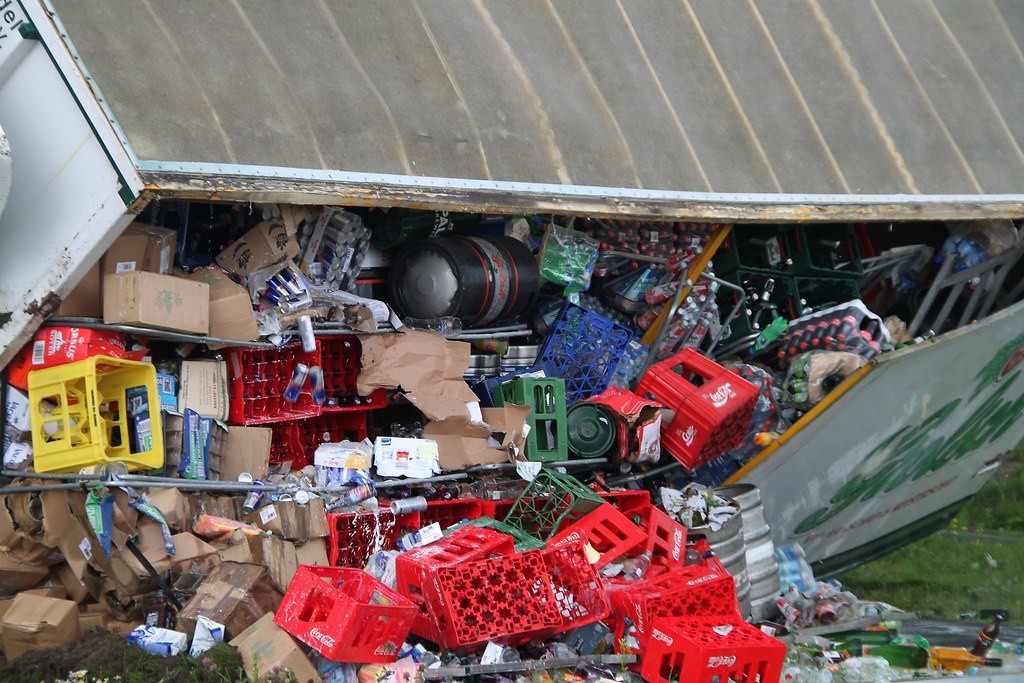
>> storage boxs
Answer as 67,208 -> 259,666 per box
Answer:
0,201 -> 977,683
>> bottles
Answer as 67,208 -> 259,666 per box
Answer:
936,217 -> 1019,295
327,483 -> 375,526
970,613 -> 1004,657
980,659 -> 1001,666
749,543 -> 929,683
532,217 -> 719,388
624,549 -> 652,581
623,616 -> 636,635
376,450 -> 718,503
684,549 -> 716,566
717,259 -> 934,486
146,589 -> 175,630
